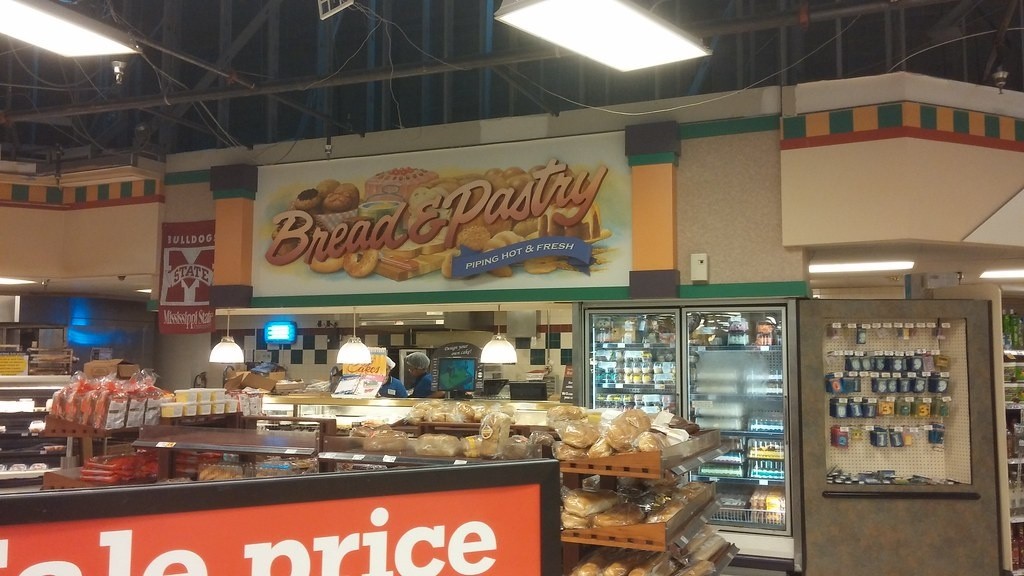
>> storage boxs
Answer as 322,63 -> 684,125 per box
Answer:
82,358 -> 138,381
225,370 -> 283,392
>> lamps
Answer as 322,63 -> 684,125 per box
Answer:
0,0 -> 143,58
479,304 -> 518,364
208,311 -> 244,363
494,0 -> 714,73
336,308 -> 372,364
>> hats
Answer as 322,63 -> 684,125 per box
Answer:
386,355 -> 396,370
404,352 -> 430,369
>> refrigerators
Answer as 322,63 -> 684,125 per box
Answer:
571,299 -> 805,575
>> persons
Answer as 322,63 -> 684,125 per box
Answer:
406,352 -> 433,398
375,355 -> 408,398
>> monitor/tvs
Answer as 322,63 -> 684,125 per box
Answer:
474,379 -> 508,395
509,381 -> 548,402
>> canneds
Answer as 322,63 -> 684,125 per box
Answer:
597,357 -> 676,409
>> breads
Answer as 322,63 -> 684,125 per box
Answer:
52,381 -> 165,428
200,402 -> 727,576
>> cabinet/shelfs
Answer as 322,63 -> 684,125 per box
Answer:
0,388 -> 559,493
560,428 -> 741,576
